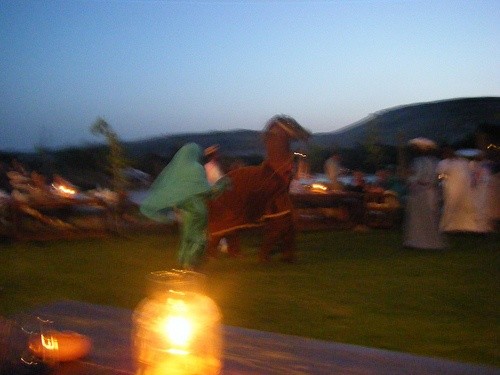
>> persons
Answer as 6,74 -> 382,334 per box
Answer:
437,147 -> 500,248
139,142 -> 234,280
285,151 -> 403,236
201,144 -> 231,256
0,166 -> 139,243
257,115 -> 312,266
404,137 -> 449,256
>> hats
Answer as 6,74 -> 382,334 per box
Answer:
408,137 -> 436,150
204,145 -> 218,156
